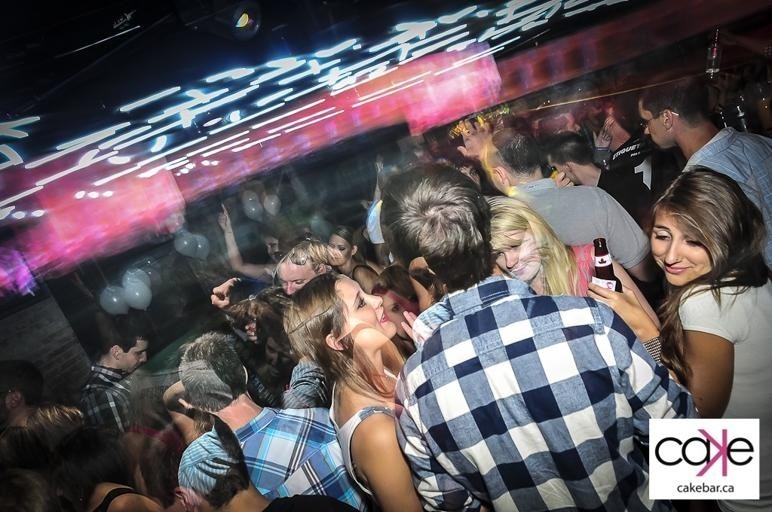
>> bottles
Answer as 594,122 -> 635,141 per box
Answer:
593,237 -> 623,296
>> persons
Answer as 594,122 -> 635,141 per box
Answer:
0,53 -> 771,510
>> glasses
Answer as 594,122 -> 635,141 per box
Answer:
639,109 -> 680,133
274,252 -> 308,266
